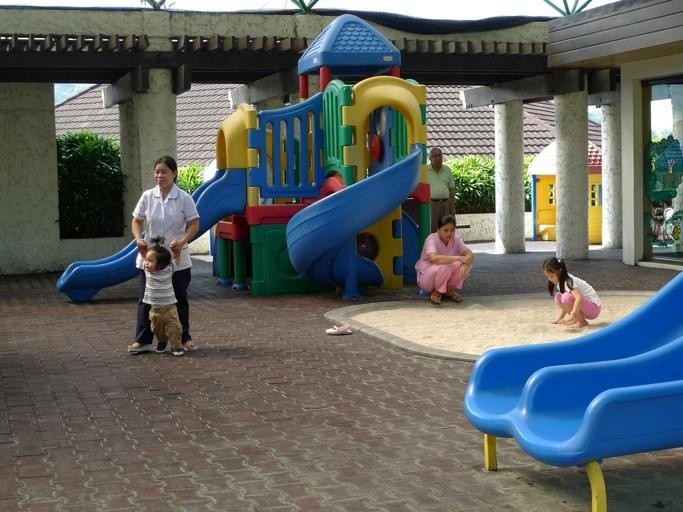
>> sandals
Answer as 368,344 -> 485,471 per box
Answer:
429,289 -> 463,305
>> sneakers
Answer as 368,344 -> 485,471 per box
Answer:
325,324 -> 353,335
127,340 -> 199,356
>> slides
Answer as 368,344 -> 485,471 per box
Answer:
56,169 -> 247,304
464,270 -> 683,467
285,146 -> 421,300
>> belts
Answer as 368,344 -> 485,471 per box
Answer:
431,198 -> 449,203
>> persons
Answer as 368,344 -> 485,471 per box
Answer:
136,238 -> 193,356
411,214 -> 475,306
539,255 -> 602,329
128,155 -> 200,354
425,147 -> 456,234
